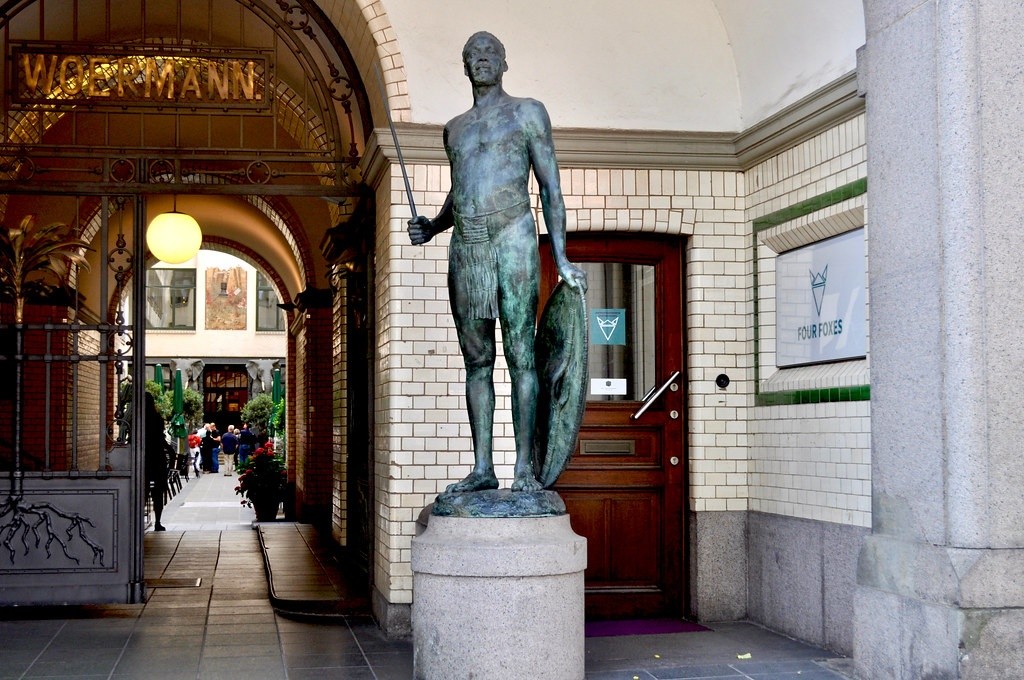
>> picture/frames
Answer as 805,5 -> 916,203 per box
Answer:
775,224 -> 866,368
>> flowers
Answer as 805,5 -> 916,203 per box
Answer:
234,441 -> 288,509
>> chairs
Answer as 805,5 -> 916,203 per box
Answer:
148,451 -> 199,513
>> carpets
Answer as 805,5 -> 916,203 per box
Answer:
584,618 -> 714,638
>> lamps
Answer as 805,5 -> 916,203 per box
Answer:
146,90 -> 202,264
217,282 -> 229,301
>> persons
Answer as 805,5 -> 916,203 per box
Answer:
407,30 -> 588,497
144,389 -> 168,532
188,422 -> 269,476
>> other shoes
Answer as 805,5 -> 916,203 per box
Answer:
155,523 -> 165,532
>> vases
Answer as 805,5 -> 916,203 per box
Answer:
253,498 -> 278,522
